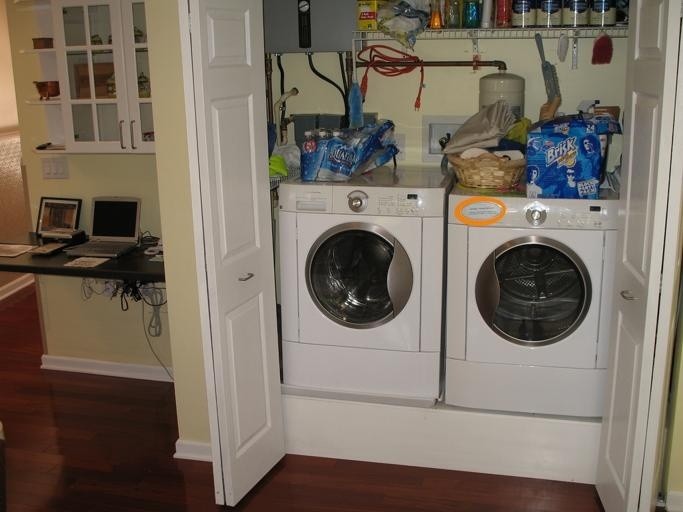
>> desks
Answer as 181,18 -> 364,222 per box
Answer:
0,240 -> 165,282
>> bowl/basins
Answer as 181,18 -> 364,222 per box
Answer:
32,37 -> 54,49
34,81 -> 60,100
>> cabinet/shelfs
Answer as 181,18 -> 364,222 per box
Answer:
13,0 -> 156,154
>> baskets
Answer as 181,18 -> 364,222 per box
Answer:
445,151 -> 528,190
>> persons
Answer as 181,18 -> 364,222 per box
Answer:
582,137 -> 596,153
526,166 -> 542,199
552,168 -> 581,200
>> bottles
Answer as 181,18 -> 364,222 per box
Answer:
348,80 -> 363,127
429,0 -> 617,29
302,127 -> 345,152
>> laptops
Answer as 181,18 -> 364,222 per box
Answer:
65,195 -> 141,258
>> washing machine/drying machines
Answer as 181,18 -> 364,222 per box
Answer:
446,184 -> 617,419
276,165 -> 456,407
